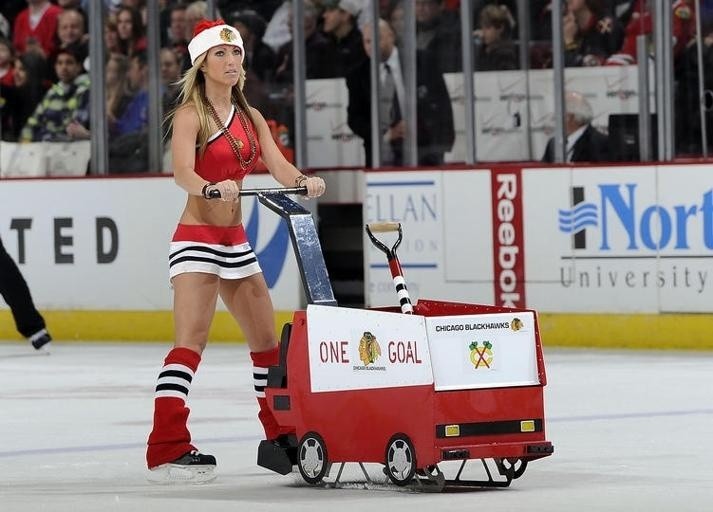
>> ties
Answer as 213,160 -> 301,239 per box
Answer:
383,63 -> 404,166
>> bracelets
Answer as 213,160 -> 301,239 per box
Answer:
295,175 -> 308,187
201,182 -> 215,200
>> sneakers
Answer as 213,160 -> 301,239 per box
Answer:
31,331 -> 53,350
145,447 -> 217,467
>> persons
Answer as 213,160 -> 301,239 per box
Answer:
146,19 -> 326,470
0,239 -> 52,349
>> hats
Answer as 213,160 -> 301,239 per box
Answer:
187,19 -> 245,66
320,0 -> 363,18
